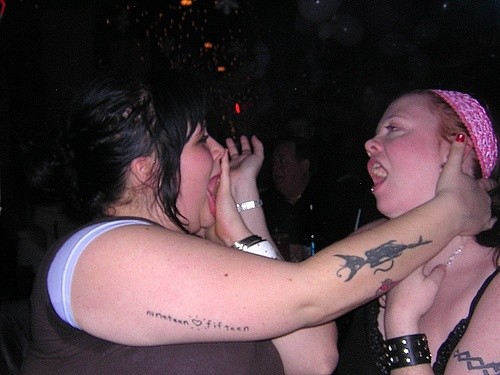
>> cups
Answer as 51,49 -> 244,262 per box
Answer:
272,234 -> 290,260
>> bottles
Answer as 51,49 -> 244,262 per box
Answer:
303,203 -> 319,260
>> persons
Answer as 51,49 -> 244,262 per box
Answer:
225,88 -> 500,375
13,62 -> 500,375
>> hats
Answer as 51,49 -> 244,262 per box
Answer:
430,87 -> 498,178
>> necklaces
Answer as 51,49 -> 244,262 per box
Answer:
443,240 -> 468,270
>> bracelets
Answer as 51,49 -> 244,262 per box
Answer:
229,235 -> 266,250
383,333 -> 432,371
237,198 -> 262,211
243,242 -> 279,259
